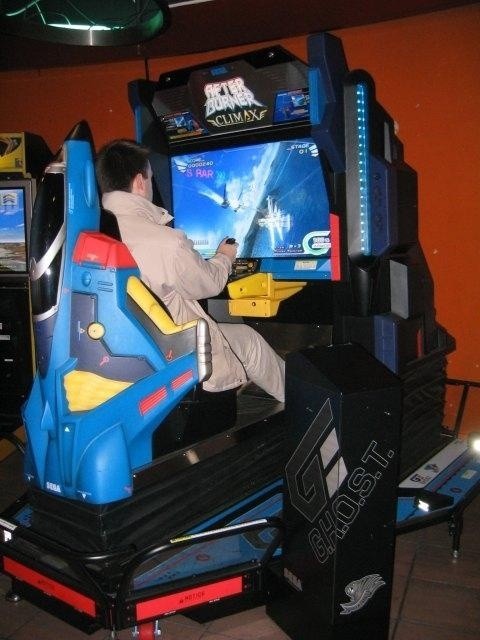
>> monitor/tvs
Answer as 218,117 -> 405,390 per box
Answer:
0,178 -> 37,278
170,136 -> 331,280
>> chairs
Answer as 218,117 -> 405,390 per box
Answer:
23,119 -> 238,505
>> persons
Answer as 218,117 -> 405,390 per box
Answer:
95,137 -> 285,403
